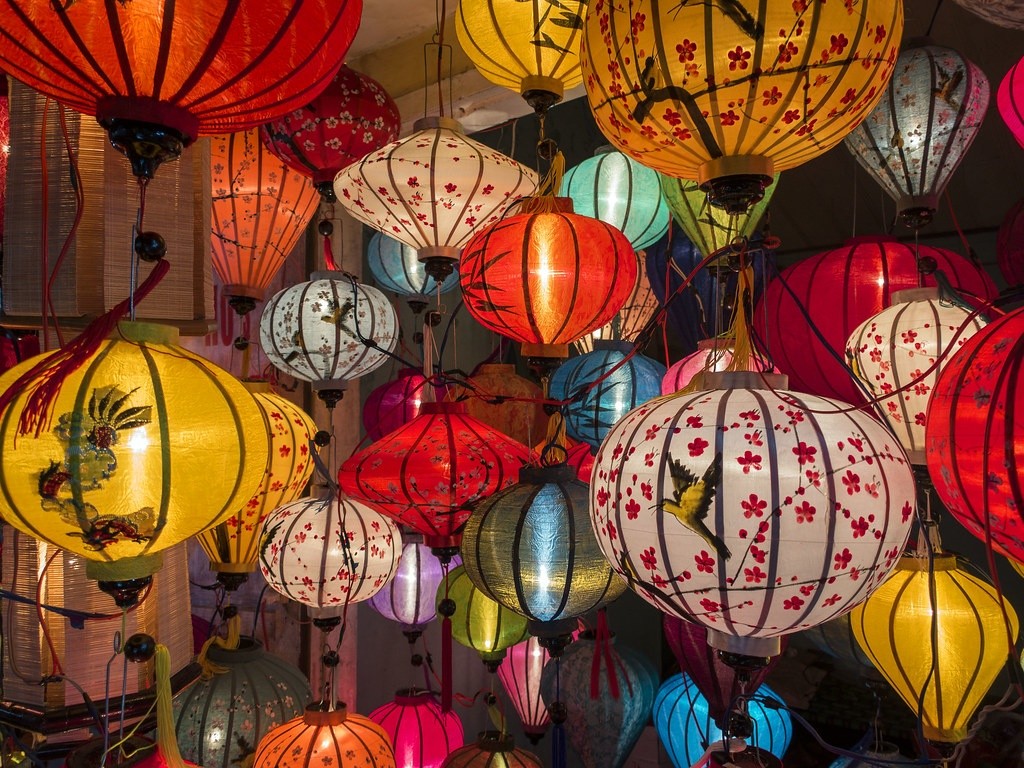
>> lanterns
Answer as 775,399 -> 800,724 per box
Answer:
0,0 -> 1024,768
